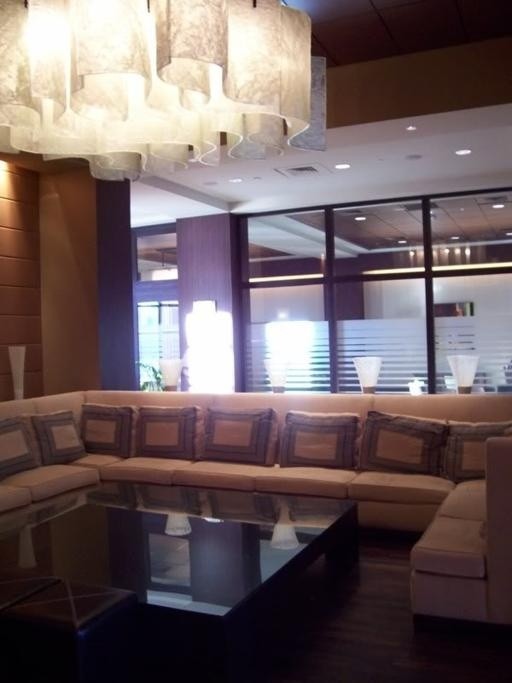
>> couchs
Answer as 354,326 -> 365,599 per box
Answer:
0,391 -> 511,633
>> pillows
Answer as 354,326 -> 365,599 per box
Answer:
0,415 -> 44,481
205,405 -> 282,467
440,417 -> 510,482
279,408 -> 363,469
361,411 -> 446,477
31,410 -> 89,464
81,402 -> 139,457
137,405 -> 207,461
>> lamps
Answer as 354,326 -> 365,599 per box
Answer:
164,507 -> 194,536
0,0 -> 325,179
352,355 -> 382,392
158,358 -> 182,391
261,354 -> 287,391
447,354 -> 480,393
269,503 -> 301,551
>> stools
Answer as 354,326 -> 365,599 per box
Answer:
0,554 -> 61,608
4,578 -> 140,682
